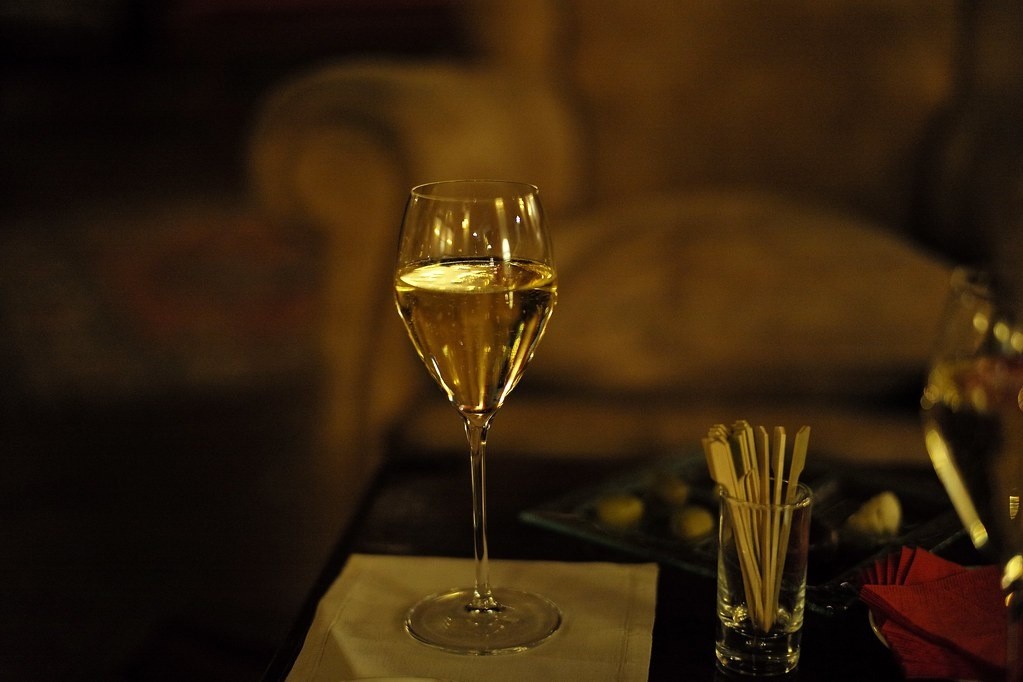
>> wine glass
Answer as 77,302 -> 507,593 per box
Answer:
917,274 -> 1021,681
392,179 -> 562,658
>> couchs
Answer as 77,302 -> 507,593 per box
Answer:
242,0 -> 1023,478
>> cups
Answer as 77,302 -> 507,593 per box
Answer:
716,479 -> 816,680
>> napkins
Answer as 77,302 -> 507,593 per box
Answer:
859,547 -> 1023,682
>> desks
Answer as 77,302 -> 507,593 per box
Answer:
266,453 -> 1000,682
